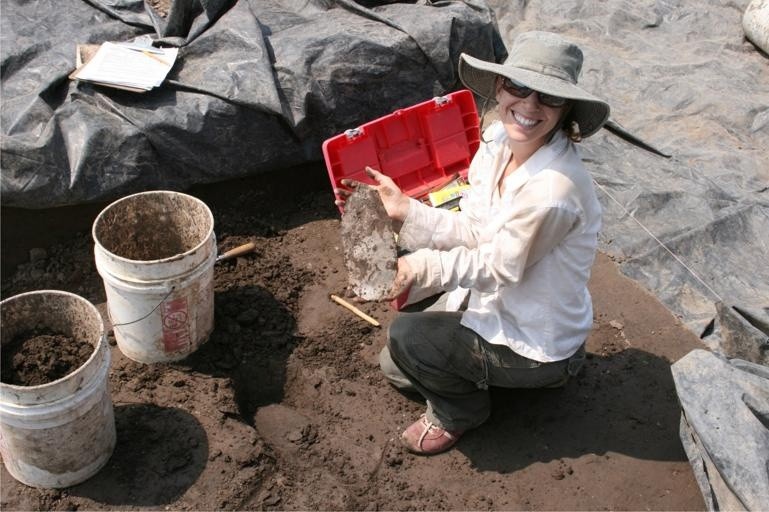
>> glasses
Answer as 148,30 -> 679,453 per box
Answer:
501,75 -> 570,108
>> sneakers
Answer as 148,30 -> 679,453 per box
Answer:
401,400 -> 491,455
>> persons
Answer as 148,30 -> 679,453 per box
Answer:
333,29 -> 611,458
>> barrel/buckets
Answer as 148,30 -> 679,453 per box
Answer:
0,289 -> 115,487
93,191 -> 218,364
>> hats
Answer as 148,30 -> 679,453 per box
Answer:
459,32 -> 610,138
